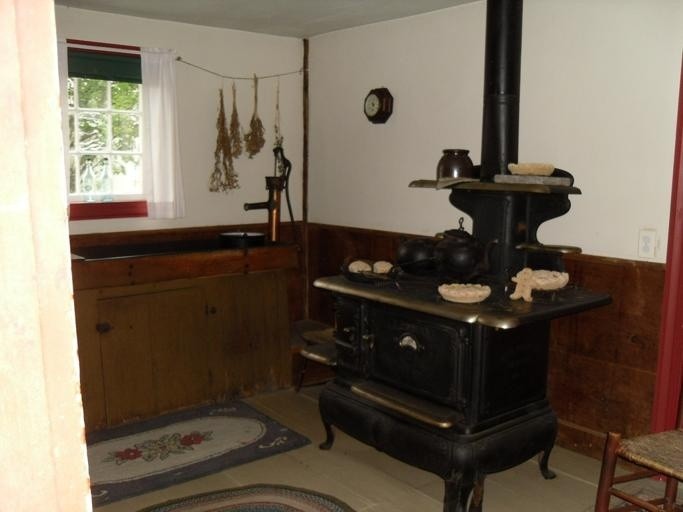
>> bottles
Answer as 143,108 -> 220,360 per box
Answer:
437,148 -> 476,178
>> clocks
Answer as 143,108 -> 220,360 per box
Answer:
364,87 -> 393,124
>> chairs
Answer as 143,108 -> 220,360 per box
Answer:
295,256 -> 376,385
73,264 -> 292,432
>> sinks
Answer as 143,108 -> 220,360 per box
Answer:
70,238 -> 281,262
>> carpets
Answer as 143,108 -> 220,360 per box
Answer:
135,483 -> 357,512
87,399 -> 311,509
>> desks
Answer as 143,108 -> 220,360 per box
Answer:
594,428 -> 683,512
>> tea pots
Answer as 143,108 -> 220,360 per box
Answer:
396,217 -> 496,280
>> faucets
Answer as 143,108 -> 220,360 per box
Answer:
245,177 -> 282,242
